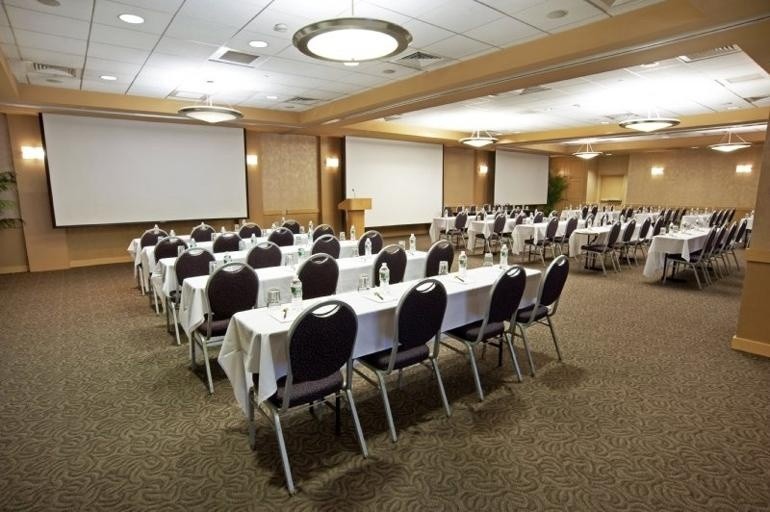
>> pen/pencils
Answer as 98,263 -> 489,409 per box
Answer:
283,308 -> 288,319
454,275 -> 465,282
373,291 -> 384,300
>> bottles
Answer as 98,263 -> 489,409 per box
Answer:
250,233 -> 256,247
669,222 -> 674,236
224,251 -> 232,264
219,225 -> 227,233
500,244 -> 507,267
484,211 -> 487,221
379,263 -> 389,291
189,238 -> 196,247
298,247 -> 305,263
603,215 -> 607,227
169,229 -> 176,237
281,216 -> 285,226
350,227 -> 355,241
308,220 -> 313,231
620,215 -> 624,225
459,251 -> 466,274
365,238 -> 371,258
587,217 -> 592,230
529,212 -> 533,224
291,275 -> 302,304
409,233 -> 416,255
444,207 -> 448,218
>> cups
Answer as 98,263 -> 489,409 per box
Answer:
660,227 -> 666,234
674,226 -> 678,232
285,253 -> 298,271
235,224 -> 240,232
398,240 -> 405,250
267,289 -> 280,309
358,273 -> 369,290
261,230 -> 267,238
340,232 -> 345,240
439,261 -> 448,275
178,245 -> 185,257
298,226 -> 305,233
271,219 -> 279,228
209,261 -> 218,274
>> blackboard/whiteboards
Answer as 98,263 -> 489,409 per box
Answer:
344,136 -> 443,230
39,112 -> 249,228
493,149 -> 550,207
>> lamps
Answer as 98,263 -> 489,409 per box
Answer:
458,123 -> 499,148
706,129 -> 752,153
291,2 -> 415,68
618,103 -> 681,133
572,139 -> 603,161
176,93 -> 245,125
